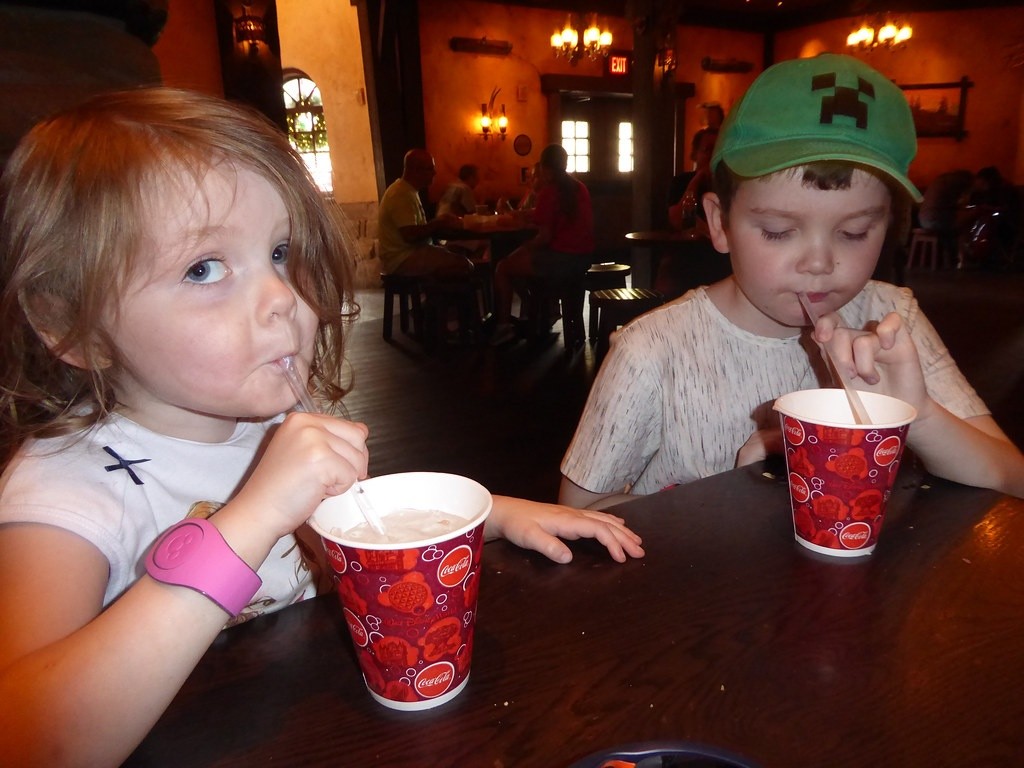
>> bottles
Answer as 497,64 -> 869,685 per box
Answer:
681,191 -> 697,240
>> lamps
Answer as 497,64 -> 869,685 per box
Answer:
481,104 -> 492,141
549,10 -> 578,63
235,16 -> 267,54
583,11 -> 613,61
497,103 -> 508,142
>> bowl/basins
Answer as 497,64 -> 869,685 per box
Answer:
570,742 -> 756,768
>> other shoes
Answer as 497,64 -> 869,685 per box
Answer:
488,325 -> 513,345
443,328 -> 482,345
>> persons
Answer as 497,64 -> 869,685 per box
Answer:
0,88 -> 645,767
378,144 -> 594,346
668,105 -> 733,287
920,165 -> 1000,269
878,193 -> 912,272
562,51 -> 1024,511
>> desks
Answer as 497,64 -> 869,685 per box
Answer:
625,230 -> 705,296
112,413 -> 1024,768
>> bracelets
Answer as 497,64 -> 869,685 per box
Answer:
145,514 -> 261,617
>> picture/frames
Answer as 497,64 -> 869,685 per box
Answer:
892,75 -> 975,141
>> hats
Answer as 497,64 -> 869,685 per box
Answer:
711,52 -> 924,203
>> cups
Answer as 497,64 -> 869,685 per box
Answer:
304,471 -> 495,713
476,205 -> 488,216
772,388 -> 917,557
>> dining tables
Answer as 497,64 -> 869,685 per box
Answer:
435,213 -> 540,330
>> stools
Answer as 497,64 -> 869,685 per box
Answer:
539,263 -> 586,347
380,273 -> 423,342
907,228 -> 940,273
634,296 -> 675,313
587,264 -> 632,293
589,287 -> 664,346
